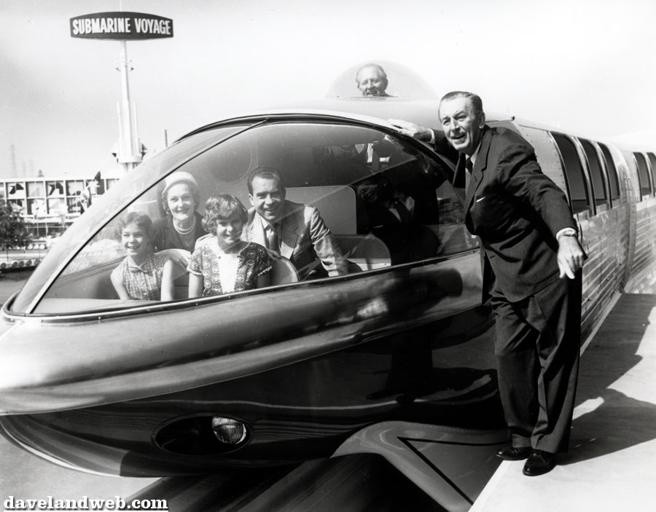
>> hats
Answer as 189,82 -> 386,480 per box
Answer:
162,171 -> 198,194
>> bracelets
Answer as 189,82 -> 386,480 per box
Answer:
555,227 -> 576,242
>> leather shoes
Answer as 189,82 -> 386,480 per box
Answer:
495,444 -> 557,476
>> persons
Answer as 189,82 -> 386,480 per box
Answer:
236,164 -> 349,283
183,193 -> 273,299
109,212 -> 175,304
386,90 -> 587,477
354,63 -> 394,98
149,171 -> 209,255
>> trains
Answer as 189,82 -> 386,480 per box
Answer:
0,102 -> 654,512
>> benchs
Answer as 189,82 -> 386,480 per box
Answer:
153,248 -> 301,300
427,223 -> 471,254
336,234 -> 392,270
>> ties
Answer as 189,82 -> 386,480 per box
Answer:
268,224 -> 278,251
465,158 -> 471,198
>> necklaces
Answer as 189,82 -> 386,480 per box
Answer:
171,214 -> 197,236
